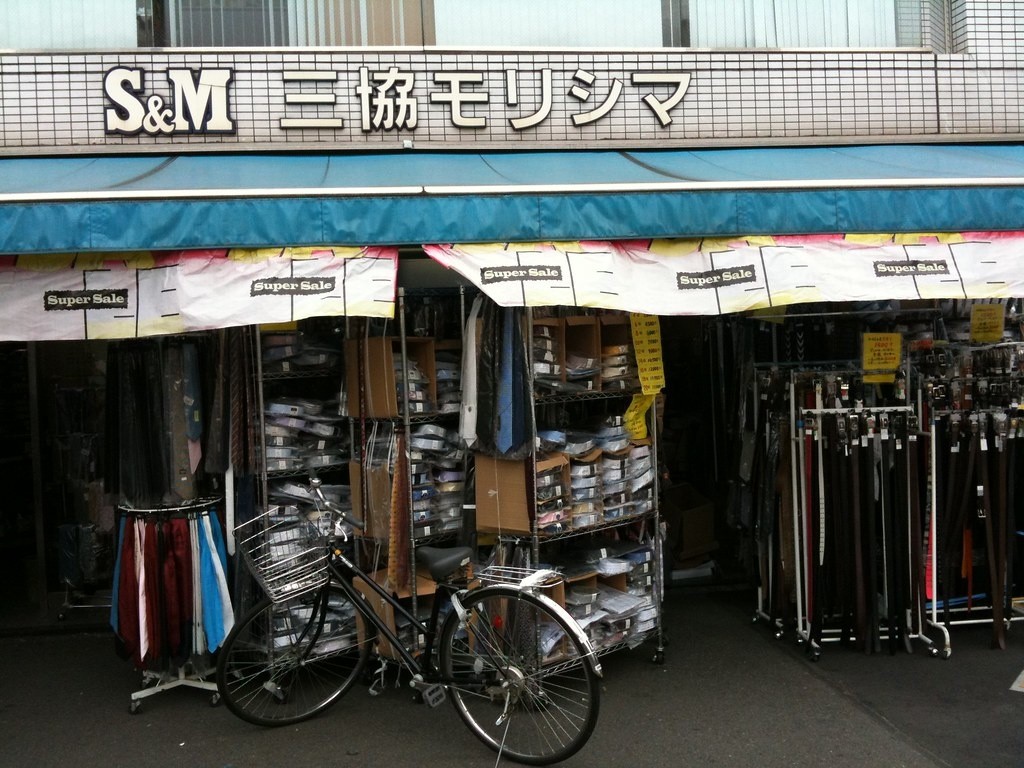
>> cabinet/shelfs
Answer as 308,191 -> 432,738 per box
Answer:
241,323 -> 358,704
459,286 -> 666,711
344,287 -> 467,705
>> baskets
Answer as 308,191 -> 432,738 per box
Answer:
231,505 -> 330,604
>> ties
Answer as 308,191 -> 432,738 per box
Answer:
459,293 -> 537,453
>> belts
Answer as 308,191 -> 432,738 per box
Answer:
737,305 -> 1024,658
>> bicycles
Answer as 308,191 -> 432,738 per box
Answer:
216,466 -> 604,768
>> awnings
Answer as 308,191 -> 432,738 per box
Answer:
0,245 -> 398,342
425,231 -> 1024,315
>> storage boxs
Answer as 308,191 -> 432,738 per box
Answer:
343,315 -> 651,661
661,485 -> 720,570
